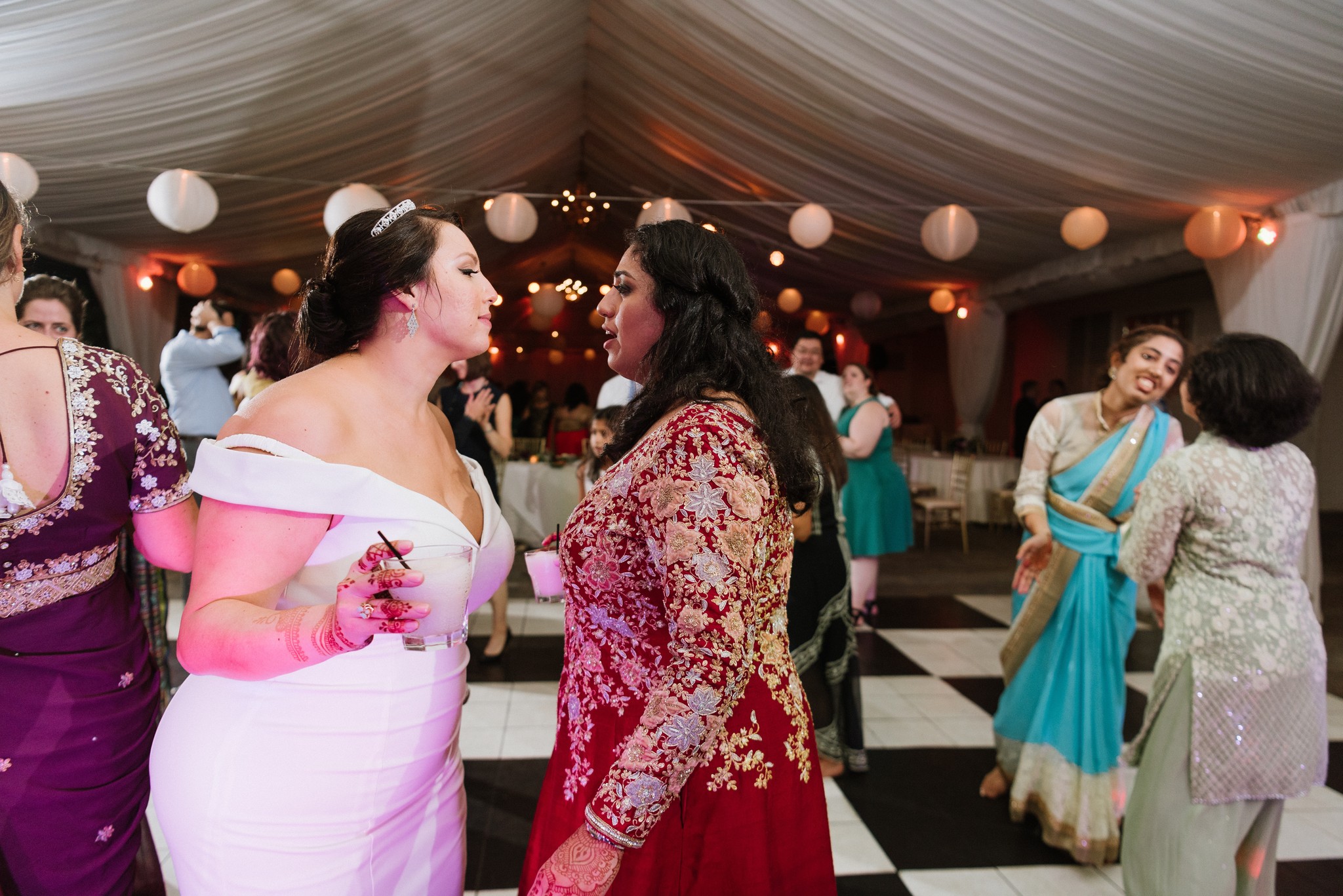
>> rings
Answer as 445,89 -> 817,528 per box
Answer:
357,597 -> 376,620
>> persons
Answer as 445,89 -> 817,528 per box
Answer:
977,322 -> 1203,872
0,182 -> 208,896
774,327 -> 901,433
226,308 -> 305,413
756,371 -> 871,780
158,294 -> 248,508
434,349 -> 516,668
520,217 -> 839,896
1107,324 -> 1331,896
576,403 -> 631,509
12,271 -> 89,344
833,360 -> 914,628
142,189 -> 520,895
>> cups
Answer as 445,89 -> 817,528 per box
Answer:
524,547 -> 566,604
379,544 -> 475,651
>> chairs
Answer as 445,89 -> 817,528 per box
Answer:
915,450 -> 974,549
984,487 -> 1023,535
889,442 -> 936,517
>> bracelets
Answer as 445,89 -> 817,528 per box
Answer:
584,820 -> 626,850
483,428 -> 494,435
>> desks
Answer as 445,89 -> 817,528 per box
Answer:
896,446 -> 1022,528
499,460 -> 589,546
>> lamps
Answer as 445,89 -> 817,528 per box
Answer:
1060,205 -> 1109,252
636,196 -> 693,231
487,191 -> 538,243
0,151 -> 41,205
134,258 -> 971,390
1182,206 -> 1248,259
322,181 -> 394,239
788,202 -> 834,250
920,203 -> 980,260
1255,215 -> 1278,246
145,165 -> 220,234
